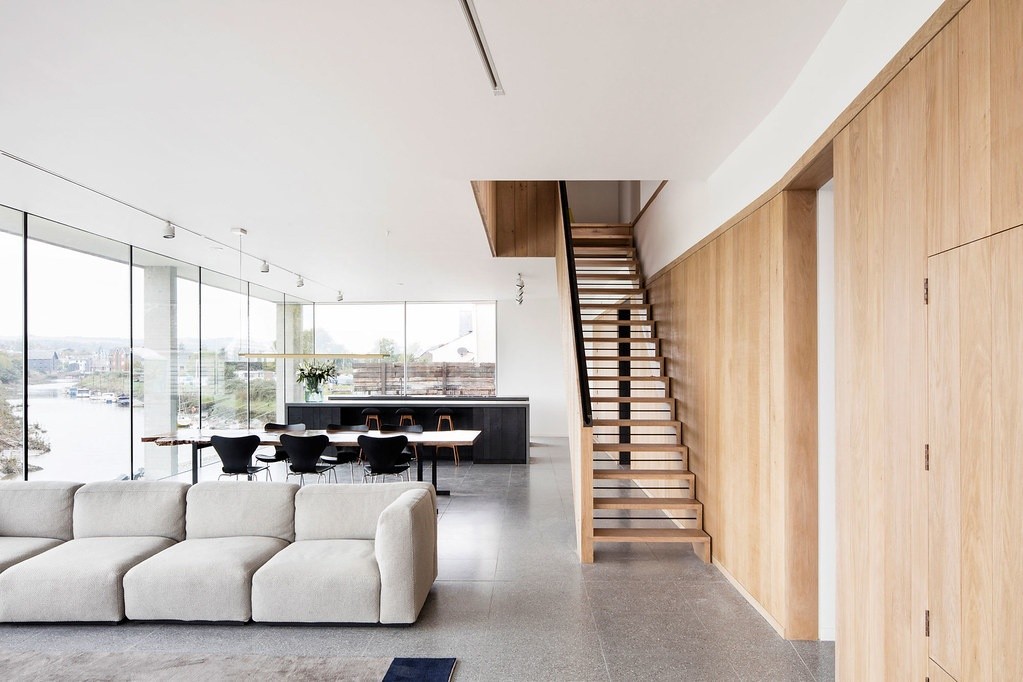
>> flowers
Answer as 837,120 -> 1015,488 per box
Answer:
295,360 -> 339,394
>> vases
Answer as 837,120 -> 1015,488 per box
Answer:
305,390 -> 323,402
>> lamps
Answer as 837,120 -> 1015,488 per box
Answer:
262,261 -> 269,272
337,292 -> 343,302
296,276 -> 305,288
162,222 -> 175,238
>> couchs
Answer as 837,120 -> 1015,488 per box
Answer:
0,480 -> 436,625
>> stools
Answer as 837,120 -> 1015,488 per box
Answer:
396,408 -> 417,462
357,408 -> 382,465
432,408 -> 460,465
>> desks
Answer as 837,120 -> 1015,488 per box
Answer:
140,430 -> 481,513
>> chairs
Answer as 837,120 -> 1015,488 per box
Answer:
211,423 -> 413,482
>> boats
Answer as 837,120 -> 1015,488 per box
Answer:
177,409 -> 191,427
76,389 -> 90,398
89,390 -> 102,400
101,393 -> 116,403
68,387 -> 78,395
117,394 -> 129,406
